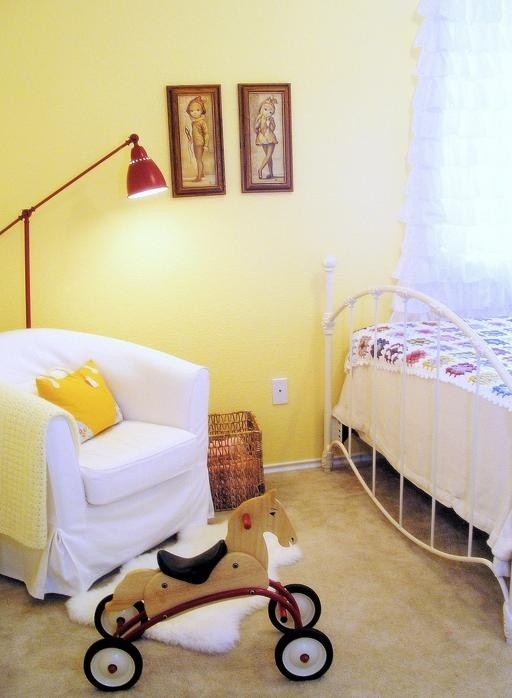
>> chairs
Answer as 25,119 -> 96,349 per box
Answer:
2,325 -> 213,602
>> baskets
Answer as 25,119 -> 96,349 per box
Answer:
207,410 -> 266,512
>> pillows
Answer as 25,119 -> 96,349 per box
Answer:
35,358 -> 124,440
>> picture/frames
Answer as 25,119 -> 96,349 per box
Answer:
165,84 -> 227,198
239,84 -> 295,196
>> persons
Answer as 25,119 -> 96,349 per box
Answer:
183,94 -> 210,184
253,95 -> 281,180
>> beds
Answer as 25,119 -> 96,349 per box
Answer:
320,251 -> 511,645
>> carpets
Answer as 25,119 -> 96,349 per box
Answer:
66,516 -> 301,655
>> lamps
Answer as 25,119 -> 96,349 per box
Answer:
0,136 -> 168,331
